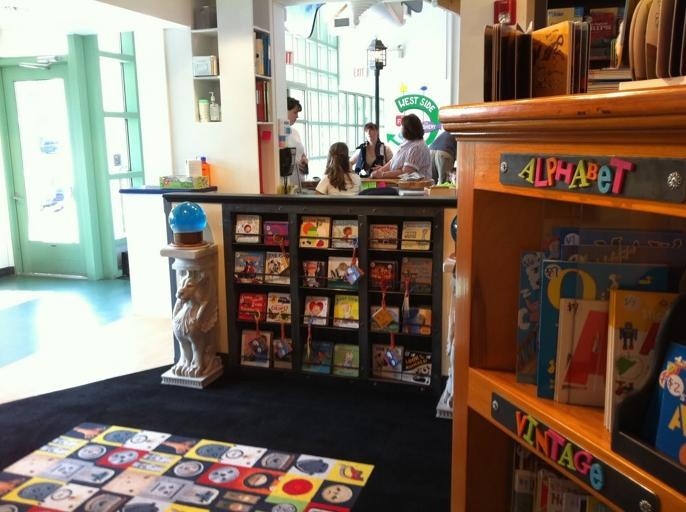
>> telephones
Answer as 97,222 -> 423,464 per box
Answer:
281,147 -> 297,176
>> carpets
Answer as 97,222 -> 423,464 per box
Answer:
0,363 -> 451,512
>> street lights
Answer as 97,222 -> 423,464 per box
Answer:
363,39 -> 390,149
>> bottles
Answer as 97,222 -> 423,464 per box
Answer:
196,157 -> 210,189
199,99 -> 210,122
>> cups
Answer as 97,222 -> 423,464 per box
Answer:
186,160 -> 202,178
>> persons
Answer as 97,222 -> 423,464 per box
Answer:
429,131 -> 457,184
288,96 -> 308,184
314,142 -> 362,195
349,120 -> 393,177
369,113 -> 433,180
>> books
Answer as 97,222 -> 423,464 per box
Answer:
253,30 -> 271,123
511,226 -> 686,511
484,0 -> 686,102
233,214 -> 433,389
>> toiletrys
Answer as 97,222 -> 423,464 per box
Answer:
201,157 -> 210,187
209,92 -> 220,122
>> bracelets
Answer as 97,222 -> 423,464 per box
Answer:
382,172 -> 385,178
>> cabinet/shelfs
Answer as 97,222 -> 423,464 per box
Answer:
253,25 -> 275,127
219,203 -> 443,396
190,27 -> 221,123
437,85 -> 686,512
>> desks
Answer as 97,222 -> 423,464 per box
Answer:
300,174 -> 434,188
